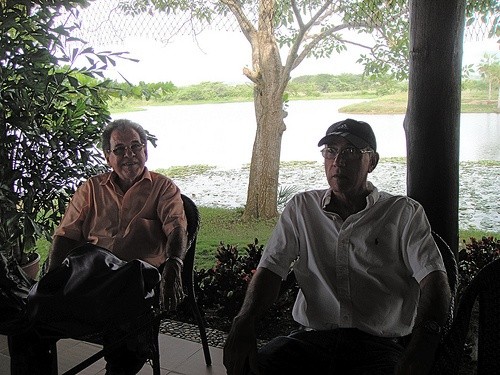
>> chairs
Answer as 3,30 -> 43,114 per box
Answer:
49,194 -> 211,375
279,231 -> 457,294
441,258 -> 500,375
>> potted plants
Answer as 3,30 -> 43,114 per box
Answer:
0,0 -> 158,280
